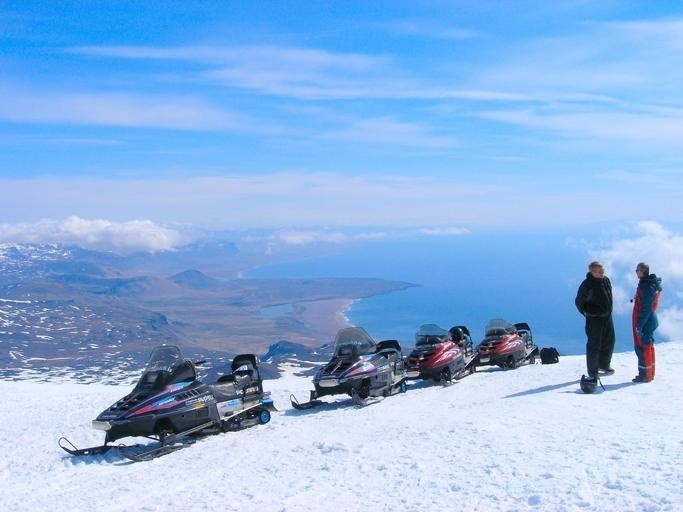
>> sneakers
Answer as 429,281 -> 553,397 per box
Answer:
632,375 -> 654,382
597,367 -> 615,375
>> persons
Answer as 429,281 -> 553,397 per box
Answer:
632,262 -> 663,382
575,261 -> 615,380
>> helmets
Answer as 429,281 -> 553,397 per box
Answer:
452,328 -> 463,340
581,374 -> 598,393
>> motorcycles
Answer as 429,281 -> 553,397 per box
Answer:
57,345 -> 278,462
290,318 -> 540,410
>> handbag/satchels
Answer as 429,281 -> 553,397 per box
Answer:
540,348 -> 559,364
